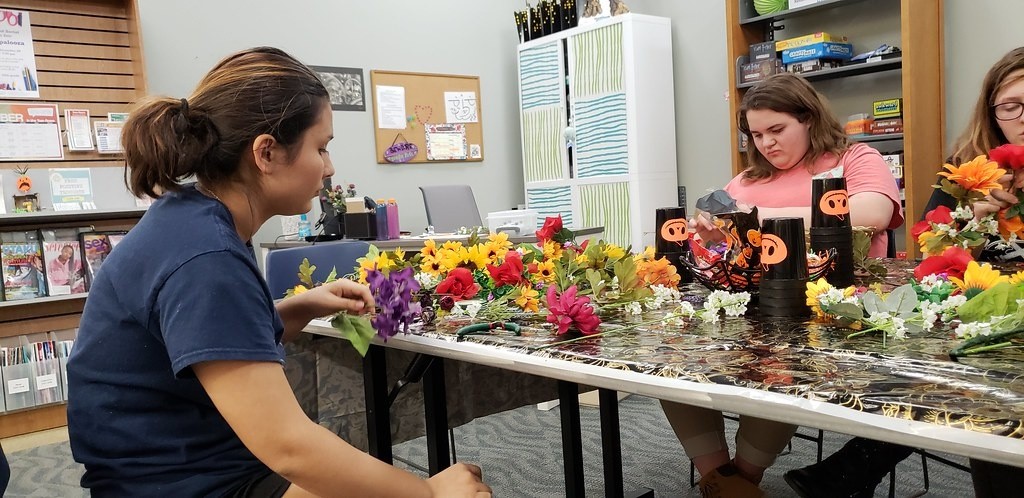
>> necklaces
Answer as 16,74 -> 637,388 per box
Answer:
194,181 -> 223,204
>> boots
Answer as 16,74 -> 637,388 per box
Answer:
780,437 -> 913,498
969,460 -> 1024,498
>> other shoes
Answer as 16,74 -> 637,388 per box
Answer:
699,460 -> 763,498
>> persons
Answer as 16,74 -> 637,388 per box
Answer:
782,46 -> 1024,497
658,72 -> 905,498
67,45 -> 493,498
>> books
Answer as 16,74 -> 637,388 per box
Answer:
0,231 -> 130,302
0,338 -> 76,414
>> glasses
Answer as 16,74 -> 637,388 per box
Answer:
992,101 -> 1024,122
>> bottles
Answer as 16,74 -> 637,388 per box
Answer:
375,200 -> 388,241
386,199 -> 400,239
297,214 -> 312,241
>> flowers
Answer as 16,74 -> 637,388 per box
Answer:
277,0 -> 1024,497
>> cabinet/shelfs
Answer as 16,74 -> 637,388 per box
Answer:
0,0 -> 151,437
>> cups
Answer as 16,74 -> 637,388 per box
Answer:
655,207 -> 693,287
759,217 -> 812,321
809,179 -> 855,289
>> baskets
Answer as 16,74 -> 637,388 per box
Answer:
487,209 -> 538,238
